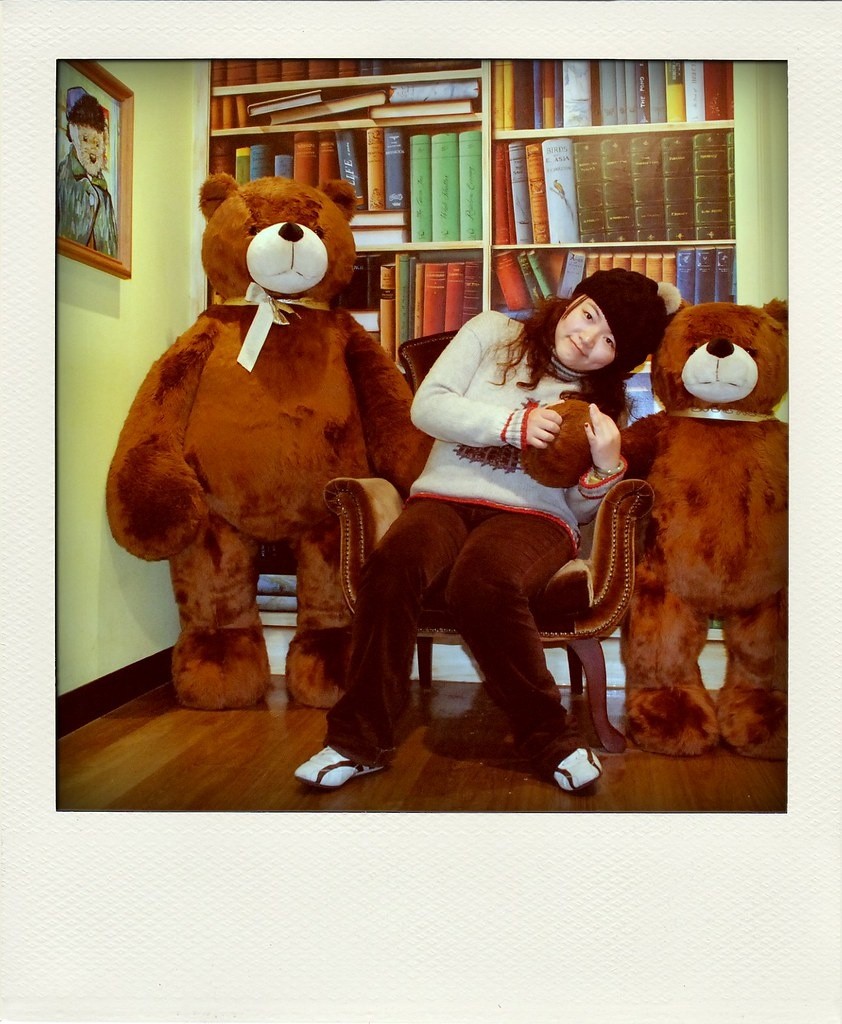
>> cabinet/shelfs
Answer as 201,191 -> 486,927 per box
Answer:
191,60 -> 772,689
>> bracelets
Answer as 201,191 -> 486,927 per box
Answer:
594,461 -> 626,474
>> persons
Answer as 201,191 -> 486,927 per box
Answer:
294,269 -> 685,789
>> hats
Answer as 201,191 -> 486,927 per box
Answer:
572,267 -> 682,372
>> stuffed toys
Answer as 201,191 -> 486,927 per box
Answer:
522,298 -> 789,760
106,171 -> 435,711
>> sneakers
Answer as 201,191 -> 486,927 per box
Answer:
294,746 -> 384,789
553,747 -> 603,791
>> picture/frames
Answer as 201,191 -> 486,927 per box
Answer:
56,58 -> 134,279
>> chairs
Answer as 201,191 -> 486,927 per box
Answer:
323,328 -> 655,754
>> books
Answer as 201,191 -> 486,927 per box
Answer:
210,59 -> 737,373
